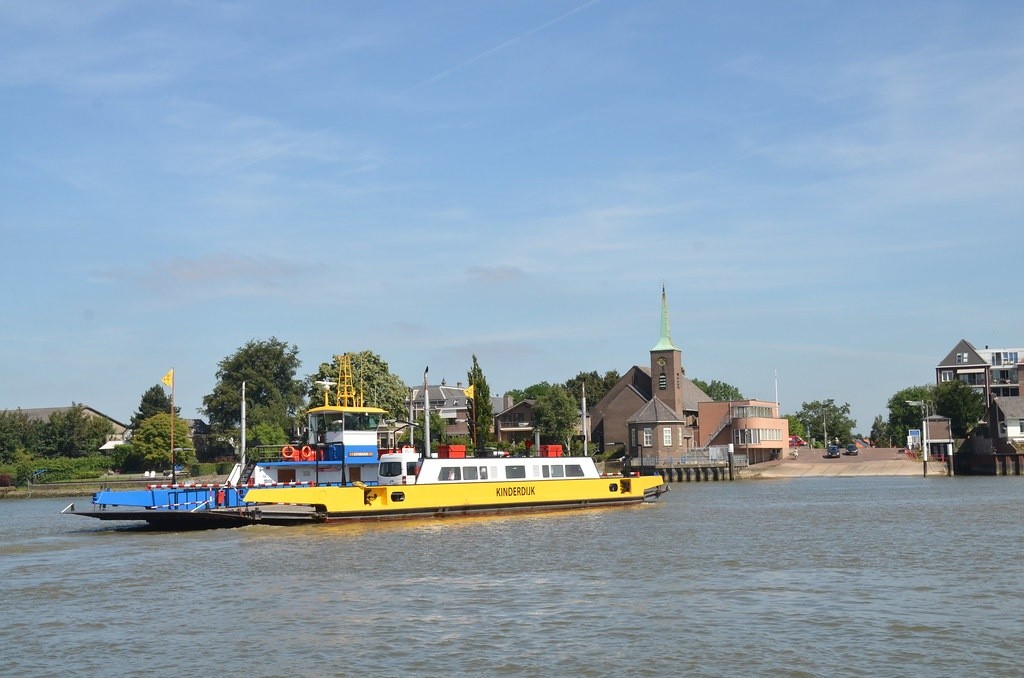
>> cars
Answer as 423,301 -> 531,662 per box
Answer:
846,444 -> 858,456
827,445 -> 841,458
789,435 -> 809,447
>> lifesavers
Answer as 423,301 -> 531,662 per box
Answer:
282,446 -> 295,458
301,446 -> 311,458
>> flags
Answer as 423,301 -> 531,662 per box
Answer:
463,385 -> 474,400
161,369 -> 174,386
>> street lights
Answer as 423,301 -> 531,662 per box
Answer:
905,399 -> 932,461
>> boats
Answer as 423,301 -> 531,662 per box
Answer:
64,352 -> 673,524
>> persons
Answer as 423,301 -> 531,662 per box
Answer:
524,438 -> 533,457
793,448 -> 799,459
